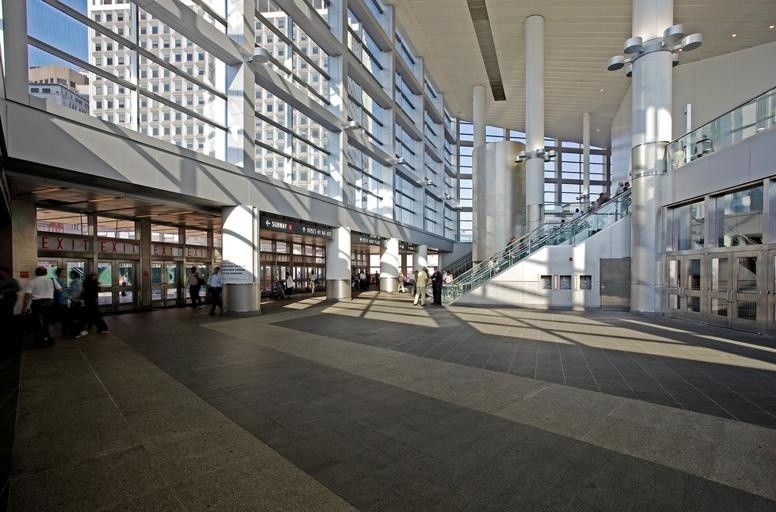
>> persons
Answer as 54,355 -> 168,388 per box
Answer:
309,271 -> 317,296
695,135 -> 715,158
488,181 -> 631,277
0,266 -> 112,357
352,268 -> 380,292
398,267 -> 454,306
287,272 -> 294,298
207,267 -> 222,316
119,272 -> 128,296
188,267 -> 204,308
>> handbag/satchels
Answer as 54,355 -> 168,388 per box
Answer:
50,278 -> 61,306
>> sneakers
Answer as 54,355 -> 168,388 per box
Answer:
76,331 -> 88,339
